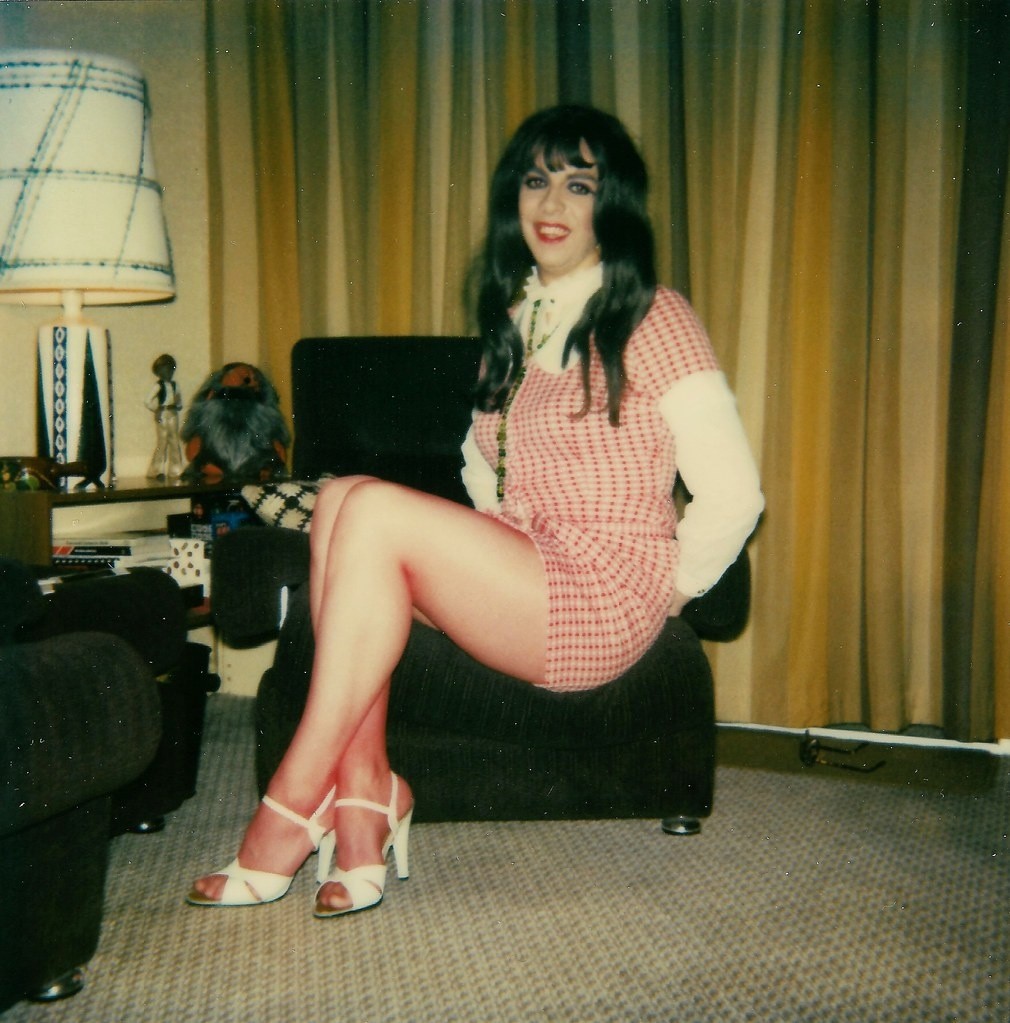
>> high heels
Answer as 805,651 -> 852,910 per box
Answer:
313,769 -> 416,918
185,784 -> 341,907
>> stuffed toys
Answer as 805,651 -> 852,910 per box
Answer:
183,361 -> 290,481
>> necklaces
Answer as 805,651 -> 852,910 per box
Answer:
494,298 -> 562,504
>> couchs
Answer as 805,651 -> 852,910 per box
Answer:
0,553 -> 163,1017
255,339 -> 753,840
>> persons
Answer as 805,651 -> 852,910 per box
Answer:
144,355 -> 186,481
182,102 -> 764,918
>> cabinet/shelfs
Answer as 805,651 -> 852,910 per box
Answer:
0,472 -> 238,636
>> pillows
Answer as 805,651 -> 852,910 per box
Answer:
241,471 -> 337,532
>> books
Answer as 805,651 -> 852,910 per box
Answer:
52,533 -> 175,568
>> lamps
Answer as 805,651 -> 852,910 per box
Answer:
0,48 -> 183,490
